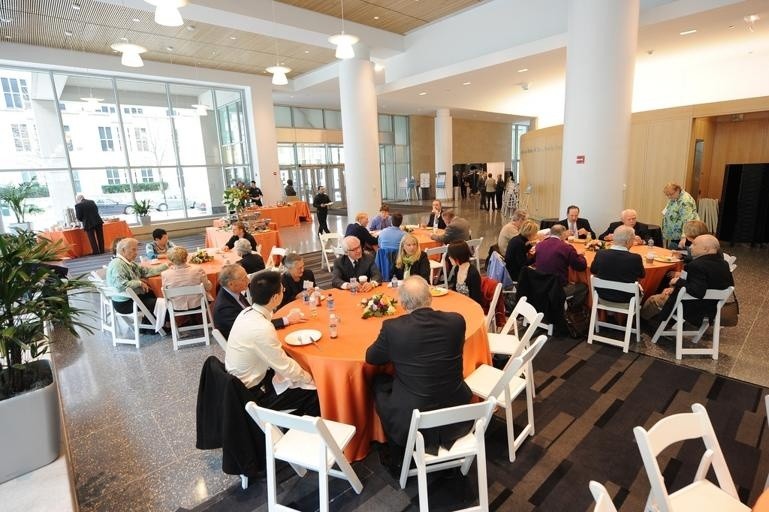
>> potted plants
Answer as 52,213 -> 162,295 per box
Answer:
0,229 -> 114,482
1,175 -> 42,234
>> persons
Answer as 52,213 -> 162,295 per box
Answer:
275,252 -> 315,312
453,166 -> 520,215
214,263 -> 306,340
223,272 -> 321,432
332,235 -> 382,293
73,194 -> 104,255
343,197 -> 473,283
284,179 -> 295,196
496,181 -> 736,341
365,276 -> 474,479
105,222 -> 266,337
443,240 -> 483,307
390,233 -> 431,283
248,181 -> 263,206
312,186 -> 331,238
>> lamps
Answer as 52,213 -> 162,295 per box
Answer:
266,50 -> 292,86
110,37 -> 147,68
329,0 -> 360,62
143,0 -> 187,27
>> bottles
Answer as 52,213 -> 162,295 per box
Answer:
586,232 -> 592,240
648,237 -> 655,249
302,286 -> 335,318
329,315 -> 338,337
392,274 -> 398,294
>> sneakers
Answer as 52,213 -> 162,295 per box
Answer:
140,318 -> 170,335
480,207 -> 501,212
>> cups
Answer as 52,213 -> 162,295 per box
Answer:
350,278 -> 357,296
647,254 -> 654,264
568,237 -> 575,244
359,276 -> 368,284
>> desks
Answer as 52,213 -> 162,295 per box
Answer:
242,201 -> 311,227
40,219 -> 134,262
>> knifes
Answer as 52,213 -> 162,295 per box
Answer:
308,335 -> 320,351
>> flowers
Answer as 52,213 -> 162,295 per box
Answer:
223,184 -> 251,213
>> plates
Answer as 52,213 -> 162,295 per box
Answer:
432,287 -> 448,296
285,330 -> 322,346
656,257 -> 682,262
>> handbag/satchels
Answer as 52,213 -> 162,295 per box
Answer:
719,302 -> 738,327
493,354 -> 509,369
565,299 -> 591,339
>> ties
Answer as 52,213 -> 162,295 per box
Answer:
570,223 -> 574,231
353,260 -> 358,272
239,297 -> 247,308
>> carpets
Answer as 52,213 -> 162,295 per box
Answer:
327,203 -> 458,216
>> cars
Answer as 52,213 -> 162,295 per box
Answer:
95,198 -> 137,216
154,194 -> 197,212
293,186 -> 306,196
